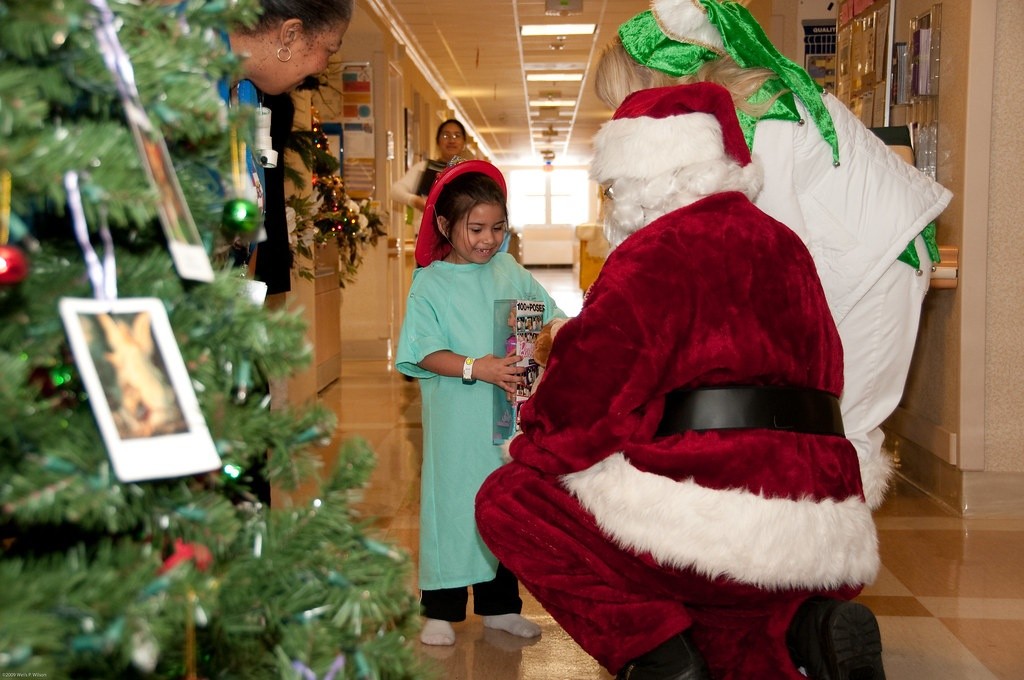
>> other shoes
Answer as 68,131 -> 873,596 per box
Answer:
785,594 -> 886,680
613,634 -> 711,680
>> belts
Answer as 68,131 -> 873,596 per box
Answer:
654,383 -> 846,439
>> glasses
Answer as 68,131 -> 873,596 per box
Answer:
604,184 -> 614,200
440,132 -> 465,140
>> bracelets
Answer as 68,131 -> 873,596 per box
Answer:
462,358 -> 477,385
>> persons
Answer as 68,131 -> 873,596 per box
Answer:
28,0 -> 352,270
593,0 -> 955,513
395,155 -> 568,646
390,119 -> 510,382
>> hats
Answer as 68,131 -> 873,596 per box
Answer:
415,155 -> 507,267
617,0 -> 839,166
589,82 -> 753,183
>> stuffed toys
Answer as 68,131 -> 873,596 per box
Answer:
475,81 -> 882,680
